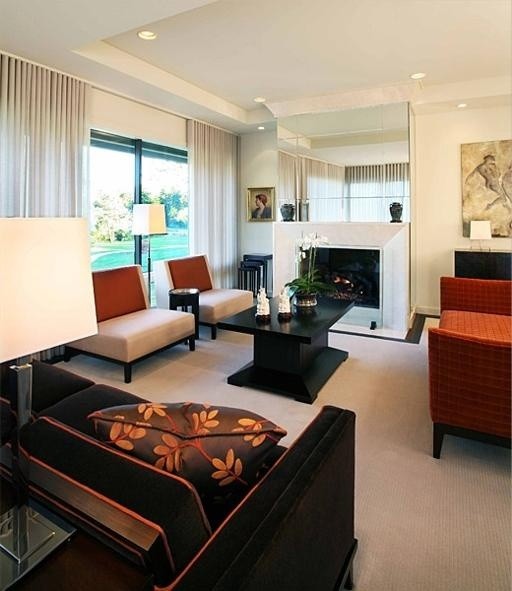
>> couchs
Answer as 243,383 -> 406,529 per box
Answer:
428,277 -> 512,459
154,255 -> 253,340
1,359 -> 358,591
64,265 -> 196,383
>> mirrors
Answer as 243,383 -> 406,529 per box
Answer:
277,102 -> 408,223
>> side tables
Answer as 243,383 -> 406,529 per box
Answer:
169,289 -> 199,351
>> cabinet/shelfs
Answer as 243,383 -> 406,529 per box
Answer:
454,248 -> 512,280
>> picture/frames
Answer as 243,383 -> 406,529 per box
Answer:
246,187 -> 275,221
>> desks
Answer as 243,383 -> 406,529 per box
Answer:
216,292 -> 356,404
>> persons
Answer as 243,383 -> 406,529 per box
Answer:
467,156 -> 508,213
251,194 -> 271,219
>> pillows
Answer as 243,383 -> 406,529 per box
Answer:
86,402 -> 287,503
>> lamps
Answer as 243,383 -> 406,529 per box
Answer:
0,216 -> 99,590
132,203 -> 167,307
470,221 -> 491,250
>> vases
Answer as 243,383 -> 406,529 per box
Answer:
294,292 -> 317,307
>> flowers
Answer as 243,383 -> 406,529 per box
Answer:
284,230 -> 343,301
